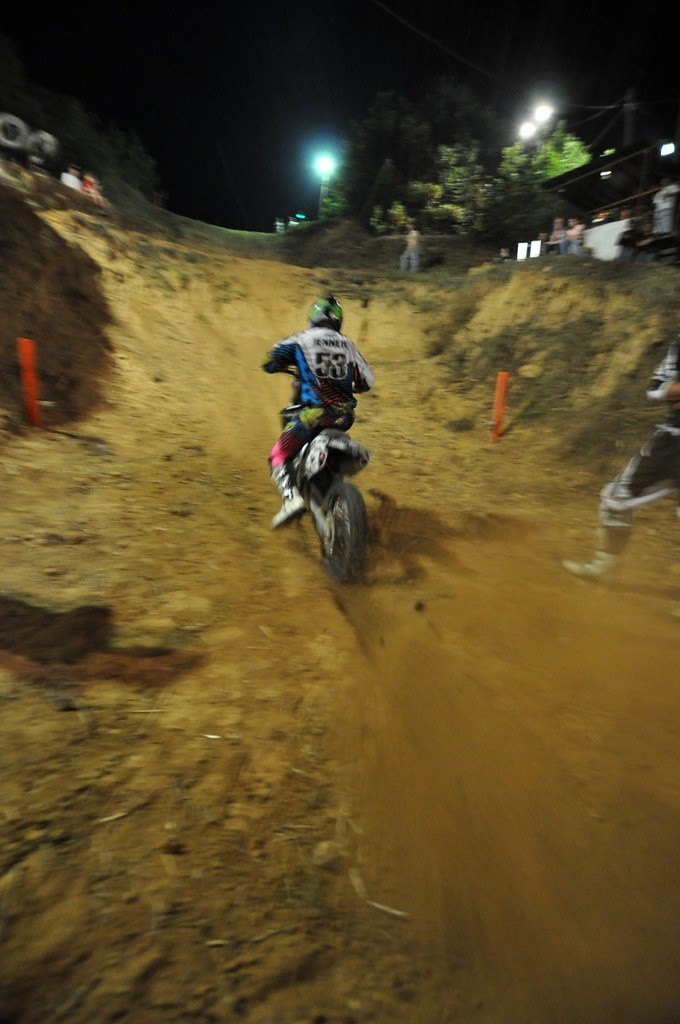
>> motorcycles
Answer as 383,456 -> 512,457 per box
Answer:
274,367 -> 372,588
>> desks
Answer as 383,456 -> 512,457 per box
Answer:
584,216 -> 640,261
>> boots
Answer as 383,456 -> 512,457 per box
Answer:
271,464 -> 304,529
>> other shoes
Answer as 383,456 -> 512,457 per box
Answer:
562,550 -> 620,589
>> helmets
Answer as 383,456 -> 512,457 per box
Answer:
307,296 -> 343,332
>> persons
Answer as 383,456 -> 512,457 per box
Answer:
536,177 -> 680,256
274,214 -> 301,233
563,333 -> 680,586
399,221 -> 424,272
262,296 -> 374,531
61,162 -> 102,195
